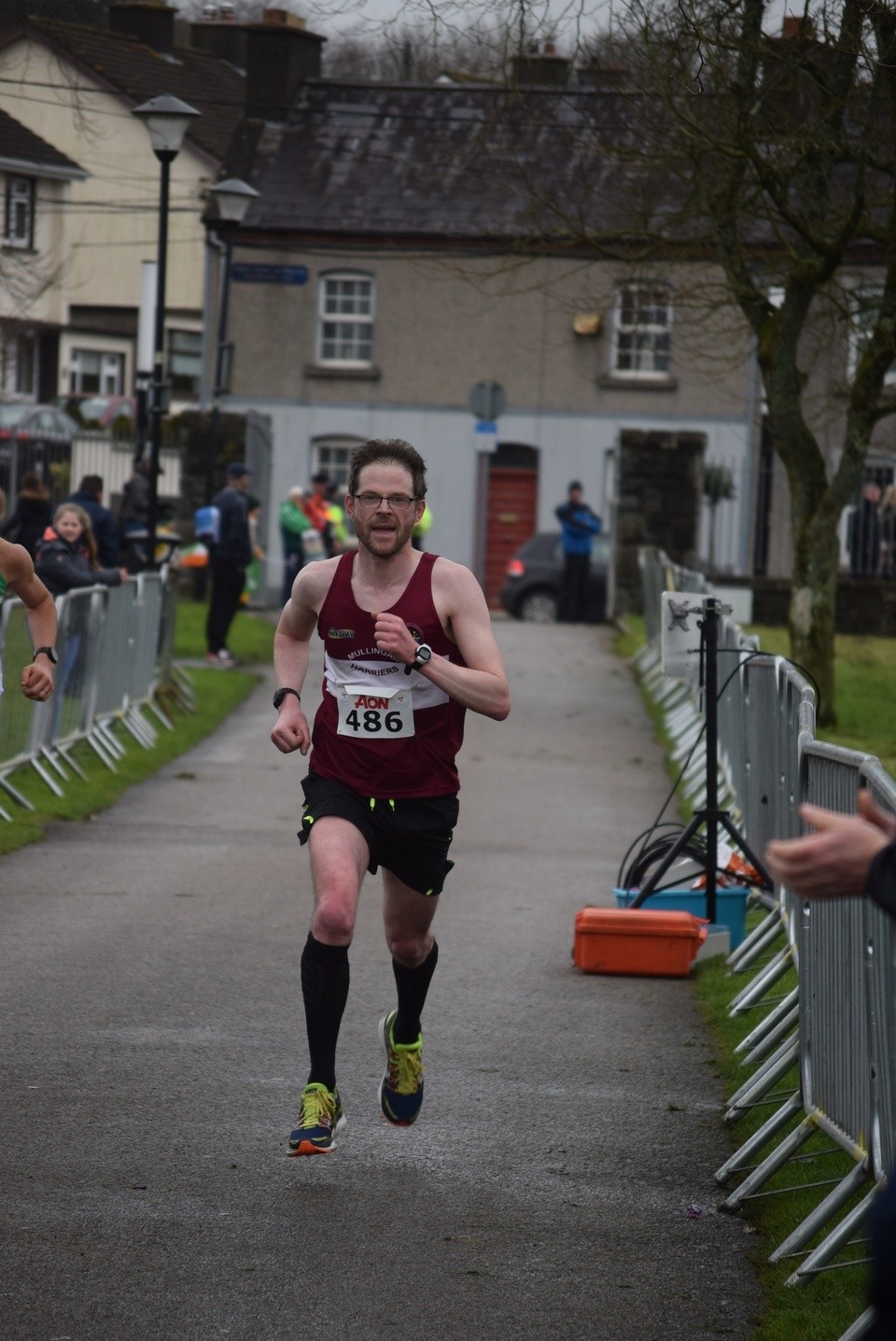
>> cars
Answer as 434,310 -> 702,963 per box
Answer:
496,530 -> 611,627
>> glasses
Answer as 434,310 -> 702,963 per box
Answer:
352,492 -> 419,510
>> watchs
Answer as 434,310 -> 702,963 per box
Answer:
32,646 -> 58,665
272,686 -> 301,710
403,642 -> 432,675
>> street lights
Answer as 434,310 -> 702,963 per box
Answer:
124,89 -> 206,574
198,176 -> 264,504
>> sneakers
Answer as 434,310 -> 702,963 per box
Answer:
378,1009 -> 424,1126
285,1083 -> 347,1157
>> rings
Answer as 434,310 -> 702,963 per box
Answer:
42,688 -> 49,694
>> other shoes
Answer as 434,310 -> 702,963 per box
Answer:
208,649 -> 239,665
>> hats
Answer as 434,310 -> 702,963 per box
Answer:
568,480 -> 582,490
226,462 -> 251,478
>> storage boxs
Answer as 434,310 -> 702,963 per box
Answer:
569,907 -> 710,977
611,883 -> 752,952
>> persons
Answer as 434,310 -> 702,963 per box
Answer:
273,471 -> 350,610
203,461 -> 251,661
0,538 -> 59,704
67,473 -> 146,573
117,458 -> 175,563
239,495 -> 265,609
269,438 -> 512,1158
411,505 -> 433,549
555,479 -> 606,623
27,502 -> 128,751
847,481 -> 885,578
764,788 -> 896,927
0,476 -> 56,559
